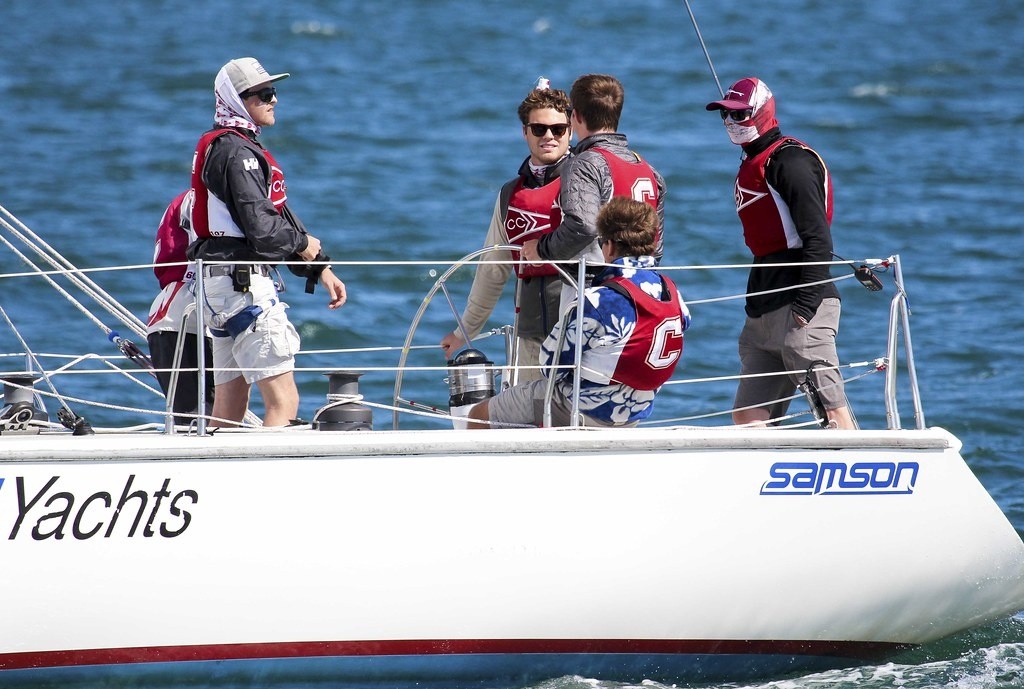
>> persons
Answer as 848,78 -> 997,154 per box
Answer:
146,56 -> 347,426
440,74 -> 692,430
704,78 -> 856,433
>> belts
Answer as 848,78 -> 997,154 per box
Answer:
200,264 -> 271,278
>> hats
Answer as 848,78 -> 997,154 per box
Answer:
705,78 -> 772,119
223,57 -> 290,94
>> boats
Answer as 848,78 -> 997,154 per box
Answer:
0,204 -> 1024,689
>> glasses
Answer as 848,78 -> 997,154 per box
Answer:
239,87 -> 276,102
598,237 -> 621,249
719,106 -> 753,121
525,122 -> 570,137
